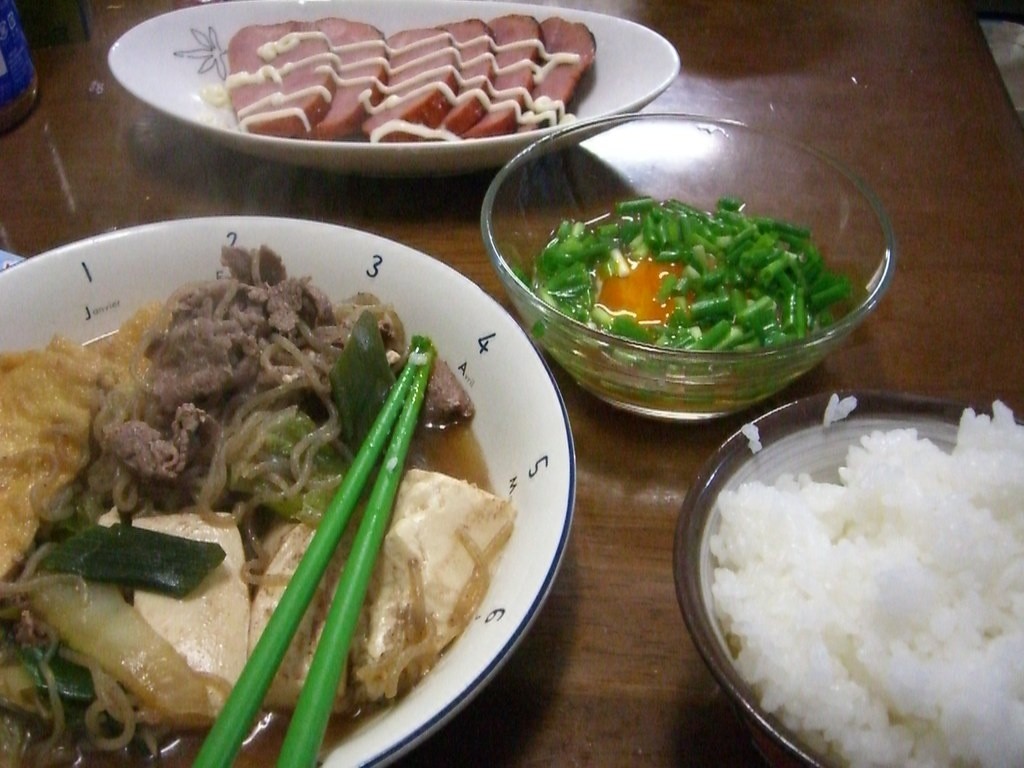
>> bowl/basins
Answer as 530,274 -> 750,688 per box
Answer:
0,216 -> 579,768
672,392 -> 1024,767
478,112 -> 895,424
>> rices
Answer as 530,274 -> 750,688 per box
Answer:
711,391 -> 1024,768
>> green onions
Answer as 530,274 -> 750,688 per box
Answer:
528,193 -> 852,404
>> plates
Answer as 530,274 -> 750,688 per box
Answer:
106,0 -> 680,177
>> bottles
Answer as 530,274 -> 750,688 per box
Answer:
0,0 -> 40,136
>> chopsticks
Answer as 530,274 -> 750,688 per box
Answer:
190,335 -> 436,768
